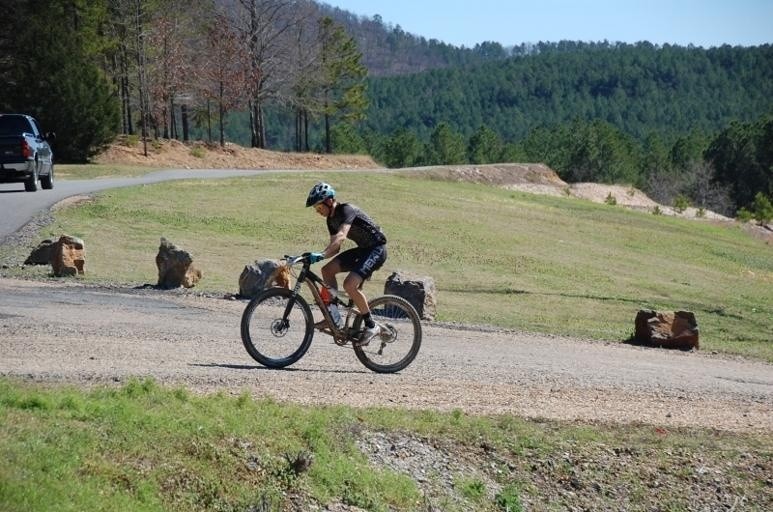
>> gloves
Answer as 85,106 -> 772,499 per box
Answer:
301,252 -> 324,264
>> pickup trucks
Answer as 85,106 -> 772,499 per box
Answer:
0,113 -> 57,192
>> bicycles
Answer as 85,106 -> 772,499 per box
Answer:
239,250 -> 423,372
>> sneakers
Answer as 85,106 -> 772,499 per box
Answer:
314,319 -> 340,328
355,323 -> 380,346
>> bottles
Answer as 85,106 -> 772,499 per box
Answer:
327,302 -> 344,331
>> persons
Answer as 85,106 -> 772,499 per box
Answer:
306,182 -> 387,347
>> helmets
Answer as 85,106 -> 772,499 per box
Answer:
306,182 -> 335,207
